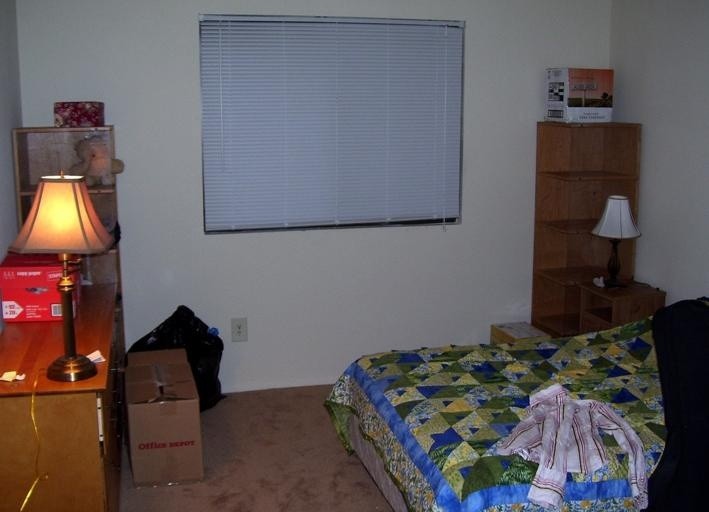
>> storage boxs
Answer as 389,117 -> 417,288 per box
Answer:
542,65 -> 614,124
2,253 -> 79,321
126,347 -> 206,488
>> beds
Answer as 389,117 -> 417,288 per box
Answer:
348,298 -> 708,512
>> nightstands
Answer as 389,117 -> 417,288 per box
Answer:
579,281 -> 667,335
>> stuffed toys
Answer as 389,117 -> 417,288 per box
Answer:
67,136 -> 124,186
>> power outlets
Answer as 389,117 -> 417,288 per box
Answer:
230,317 -> 248,343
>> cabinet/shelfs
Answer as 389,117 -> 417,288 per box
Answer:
1,282 -> 126,512
9,124 -> 122,284
530,122 -> 642,338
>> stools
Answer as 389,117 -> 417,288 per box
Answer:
490,321 -> 551,346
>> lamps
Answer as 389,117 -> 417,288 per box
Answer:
590,192 -> 640,289
7,170 -> 114,384
